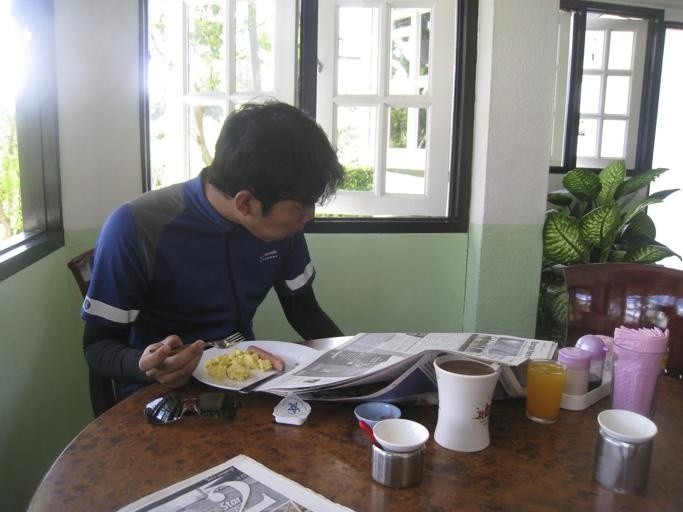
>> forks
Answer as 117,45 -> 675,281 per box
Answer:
148,332 -> 246,353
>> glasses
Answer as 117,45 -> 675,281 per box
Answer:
145,389 -> 241,426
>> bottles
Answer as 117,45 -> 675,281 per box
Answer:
557,334 -> 606,394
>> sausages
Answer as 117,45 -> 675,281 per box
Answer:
248,345 -> 286,372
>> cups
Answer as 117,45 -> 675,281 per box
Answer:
432,356 -> 500,453
526,359 -> 567,426
612,343 -> 669,419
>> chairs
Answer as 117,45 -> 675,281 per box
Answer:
563,262 -> 683,378
67,246 -> 116,419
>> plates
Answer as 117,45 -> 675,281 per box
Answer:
191,339 -> 323,392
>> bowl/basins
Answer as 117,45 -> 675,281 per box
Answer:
372,418 -> 430,453
595,409 -> 658,444
352,402 -> 402,426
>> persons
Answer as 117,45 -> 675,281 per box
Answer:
76,97 -> 346,405
